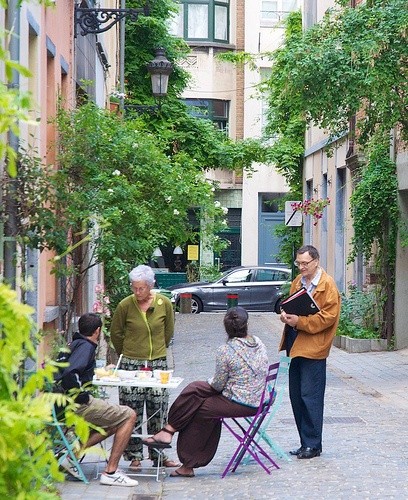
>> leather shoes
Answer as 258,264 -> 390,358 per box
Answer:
289,446 -> 322,455
297,447 -> 321,458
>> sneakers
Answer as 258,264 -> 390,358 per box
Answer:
58,455 -> 84,481
100,468 -> 139,486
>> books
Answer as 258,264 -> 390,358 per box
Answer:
280,286 -> 320,317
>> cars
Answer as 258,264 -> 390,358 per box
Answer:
150,266 -> 292,314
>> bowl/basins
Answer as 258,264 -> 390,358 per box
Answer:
154,370 -> 174,381
113,370 -> 152,380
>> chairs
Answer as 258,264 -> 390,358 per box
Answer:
24,371 -> 89,484
233,355 -> 291,466
213,362 -> 280,478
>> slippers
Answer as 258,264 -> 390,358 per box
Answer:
152,456 -> 181,467
141,437 -> 172,448
130,460 -> 142,472
169,470 -> 195,477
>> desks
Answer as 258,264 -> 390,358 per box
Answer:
91,370 -> 185,482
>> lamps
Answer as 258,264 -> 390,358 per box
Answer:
119,47 -> 173,116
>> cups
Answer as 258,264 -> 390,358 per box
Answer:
160,371 -> 169,384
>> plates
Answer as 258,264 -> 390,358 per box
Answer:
93,375 -> 122,382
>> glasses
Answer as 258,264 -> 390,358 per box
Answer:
129,284 -> 149,293
294,258 -> 319,267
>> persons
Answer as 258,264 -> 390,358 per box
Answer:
58,313 -> 138,487
142,307 -> 273,477
278,245 -> 341,460
110,264 -> 183,471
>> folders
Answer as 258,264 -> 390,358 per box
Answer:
279,287 -> 320,316
